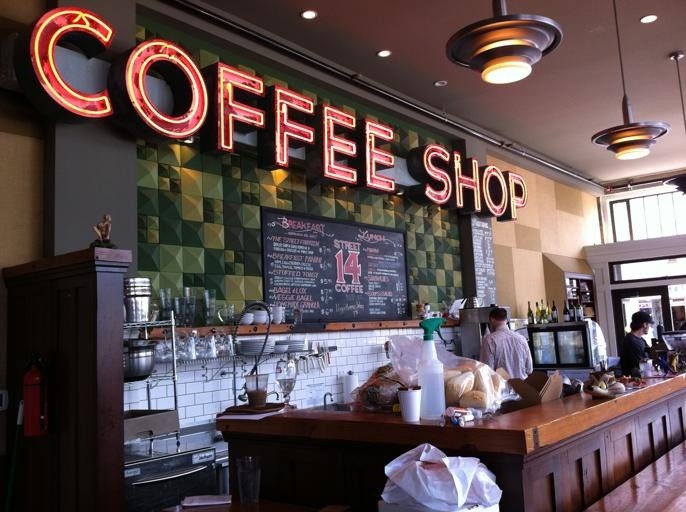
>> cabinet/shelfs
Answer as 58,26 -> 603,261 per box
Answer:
545,272 -> 599,322
118,307 -> 185,463
526,317 -> 609,371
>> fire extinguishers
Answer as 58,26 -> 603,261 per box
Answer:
22,352 -> 48,437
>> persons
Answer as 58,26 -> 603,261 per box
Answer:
620,311 -> 654,376
479,308 -> 533,381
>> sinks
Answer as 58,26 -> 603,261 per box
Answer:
135,429 -> 224,453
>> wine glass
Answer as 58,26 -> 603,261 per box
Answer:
275,360 -> 299,414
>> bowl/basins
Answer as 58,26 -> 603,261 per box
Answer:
233,305 -> 283,325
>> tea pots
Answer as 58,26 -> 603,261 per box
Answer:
460,292 -> 484,309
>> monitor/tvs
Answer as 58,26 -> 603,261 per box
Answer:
660,330 -> 686,351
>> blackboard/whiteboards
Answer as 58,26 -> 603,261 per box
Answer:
471,214 -> 498,307
259,205 -> 413,323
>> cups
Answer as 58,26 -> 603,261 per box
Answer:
152,332 -> 234,366
216,303 -> 235,325
157,284 -> 209,327
397,386 -> 422,423
243,373 -> 270,409
657,325 -> 663,338
124,277 -> 152,322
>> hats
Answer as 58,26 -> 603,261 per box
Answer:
632,312 -> 654,324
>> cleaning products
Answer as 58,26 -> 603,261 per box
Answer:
417,317 -> 446,420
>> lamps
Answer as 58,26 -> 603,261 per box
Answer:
443,1 -> 564,87
589,0 -> 671,163
662,48 -> 686,196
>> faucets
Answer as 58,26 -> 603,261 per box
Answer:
324,393 -> 334,409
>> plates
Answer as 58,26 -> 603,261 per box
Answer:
275,340 -> 306,354
234,336 -> 276,356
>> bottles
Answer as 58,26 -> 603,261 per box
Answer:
563,299 -> 583,322
534,299 -> 560,324
525,298 -> 534,325
424,303 -> 430,314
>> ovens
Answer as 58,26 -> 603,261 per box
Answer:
122,446 -> 216,511
